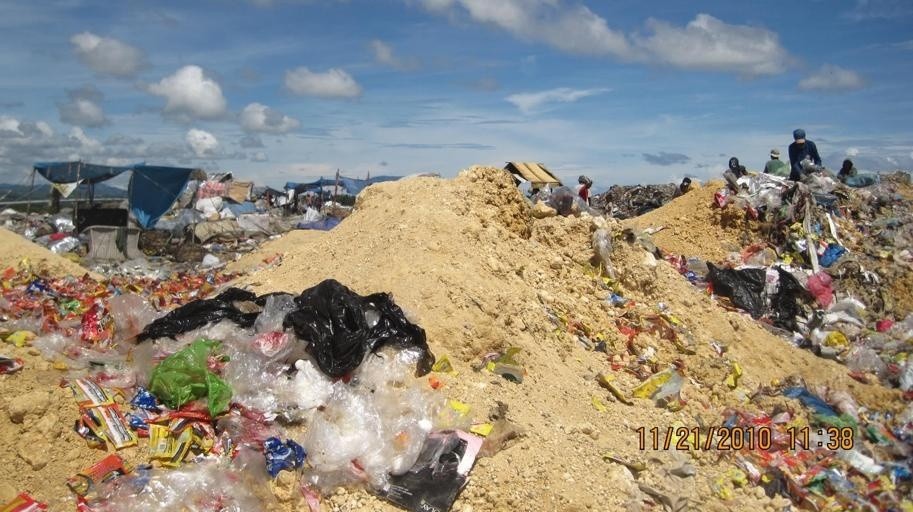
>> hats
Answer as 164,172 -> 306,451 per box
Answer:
793,128 -> 806,144
770,148 -> 781,158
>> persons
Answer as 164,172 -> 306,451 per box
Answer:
578,176 -> 592,207
576,175 -> 591,208
787,128 -> 824,183
763,148 -> 786,176
836,159 -> 857,181
